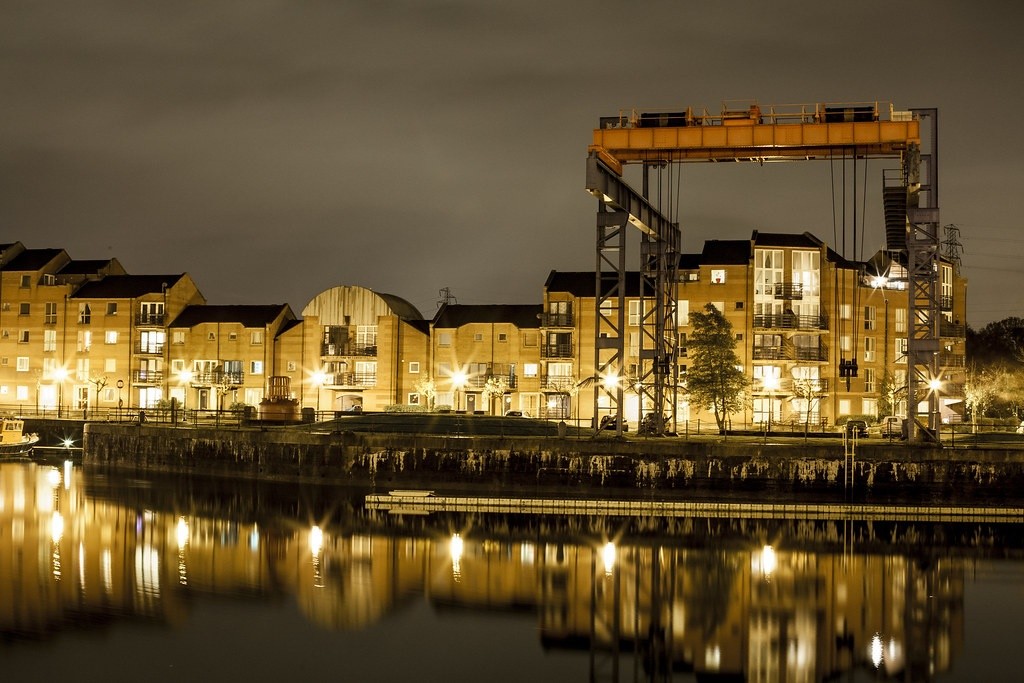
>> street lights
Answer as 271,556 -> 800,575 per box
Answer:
310,371 -> 324,422
452,371 -> 467,411
764,374 -> 777,432
181,370 -> 192,421
604,374 -> 618,416
54,367 -> 67,418
